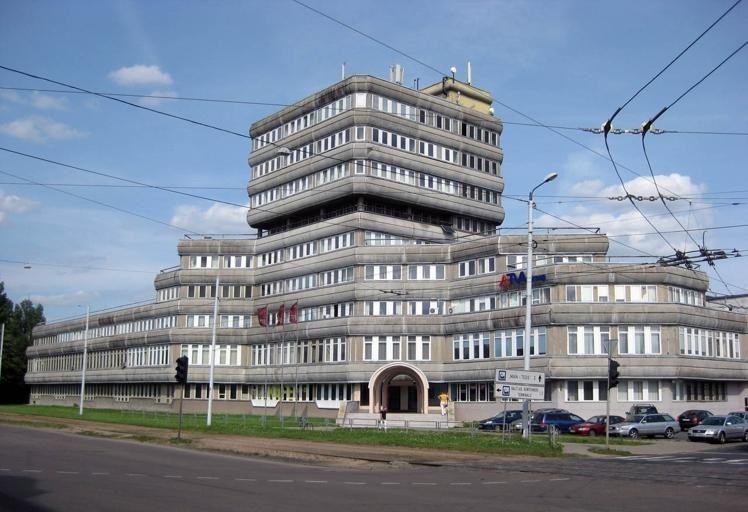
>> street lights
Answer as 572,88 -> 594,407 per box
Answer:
522,171 -> 558,440
204,236 -> 222,426
76,304 -> 89,415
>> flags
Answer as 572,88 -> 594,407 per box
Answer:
257,303 -> 298,327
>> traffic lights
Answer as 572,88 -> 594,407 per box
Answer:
610,359 -> 620,389
174,355 -> 188,383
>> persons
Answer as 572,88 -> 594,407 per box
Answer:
438,392 -> 450,415
378,406 -> 387,433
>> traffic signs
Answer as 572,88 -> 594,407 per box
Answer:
493,368 -> 545,399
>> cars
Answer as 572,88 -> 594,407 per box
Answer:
479,404 -> 748,444
